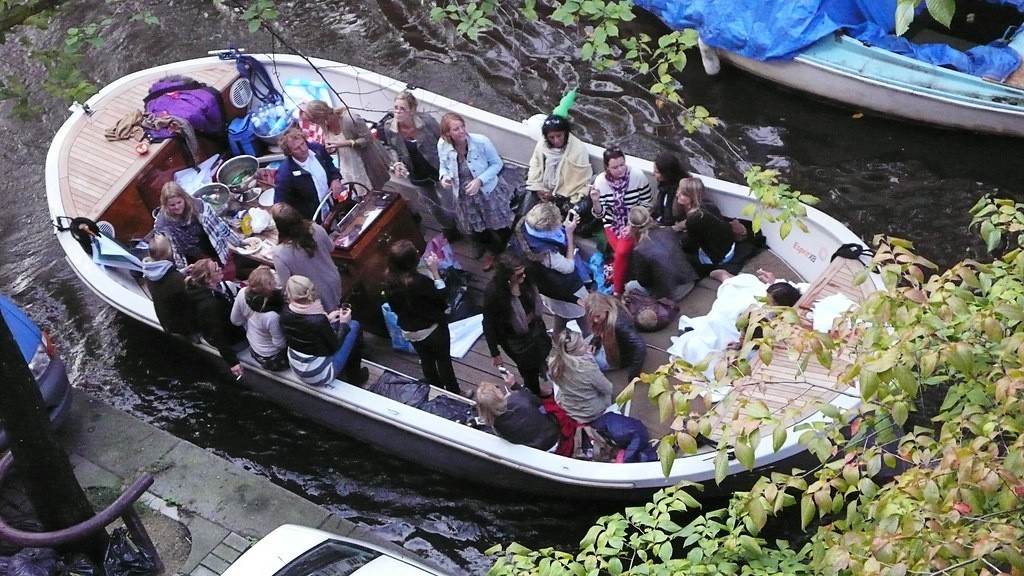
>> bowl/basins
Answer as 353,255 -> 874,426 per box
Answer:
136,143 -> 149,155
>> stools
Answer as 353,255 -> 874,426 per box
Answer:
227,125 -> 259,156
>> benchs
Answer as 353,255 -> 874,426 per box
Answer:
136,156 -> 809,427
902,26 -> 985,56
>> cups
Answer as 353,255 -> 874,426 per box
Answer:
341,303 -> 351,315
422,251 -> 434,263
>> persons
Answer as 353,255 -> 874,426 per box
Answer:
137,88 -> 809,466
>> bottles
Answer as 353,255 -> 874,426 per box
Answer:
201,193 -> 222,210
227,170 -> 248,185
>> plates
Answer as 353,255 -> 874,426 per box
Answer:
258,188 -> 275,207
237,237 -> 262,255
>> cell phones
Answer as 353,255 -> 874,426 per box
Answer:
567,209 -> 576,223
499,367 -> 507,377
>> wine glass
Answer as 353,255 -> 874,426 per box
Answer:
192,183 -> 233,226
216,155 -> 262,202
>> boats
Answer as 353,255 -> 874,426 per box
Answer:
44,46 -> 903,521
636,0 -> 1023,153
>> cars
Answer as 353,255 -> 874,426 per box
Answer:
216,523 -> 443,576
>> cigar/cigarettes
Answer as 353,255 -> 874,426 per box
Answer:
236,375 -> 241,380
